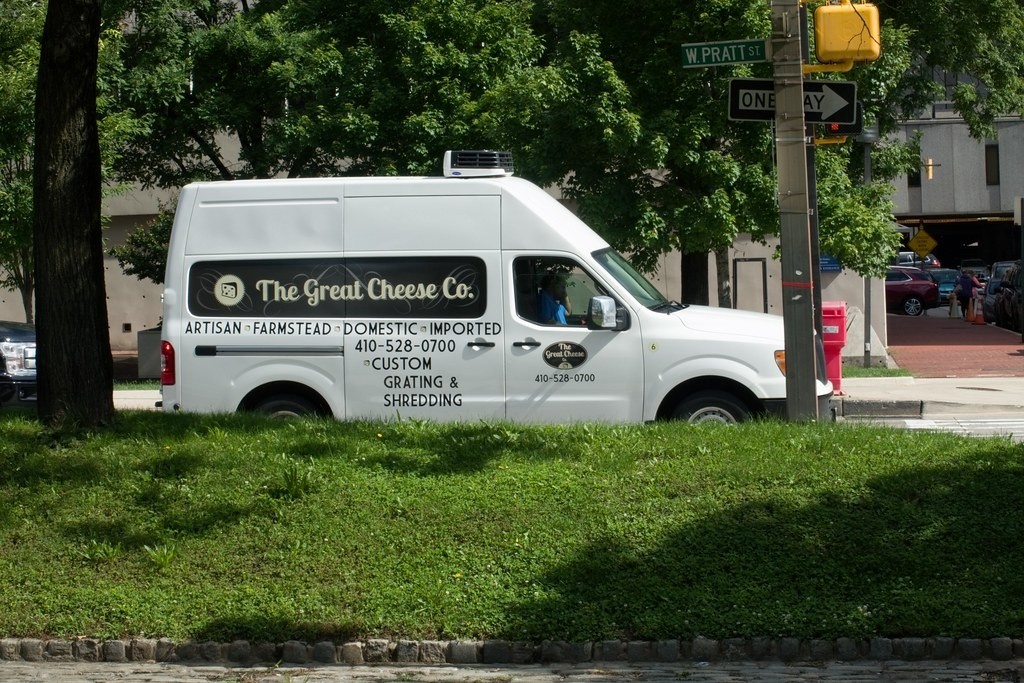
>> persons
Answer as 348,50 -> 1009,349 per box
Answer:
955,270 -> 983,319
537,277 -> 571,326
966,270 -> 986,310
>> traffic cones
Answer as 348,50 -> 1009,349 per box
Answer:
971,299 -> 988,325
963,298 -> 976,323
948,296 -> 962,320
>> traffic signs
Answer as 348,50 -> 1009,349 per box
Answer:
726,79 -> 859,125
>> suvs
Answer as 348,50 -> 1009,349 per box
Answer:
884,264 -> 942,316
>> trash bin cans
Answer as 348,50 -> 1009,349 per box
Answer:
137,330 -> 162,378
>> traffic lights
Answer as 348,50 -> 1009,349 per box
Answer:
822,99 -> 864,137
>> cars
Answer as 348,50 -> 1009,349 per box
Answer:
0,319 -> 38,407
898,250 -> 1024,335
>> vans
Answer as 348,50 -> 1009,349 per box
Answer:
154,147 -> 836,433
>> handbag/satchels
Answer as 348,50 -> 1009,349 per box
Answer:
955,275 -> 963,296
